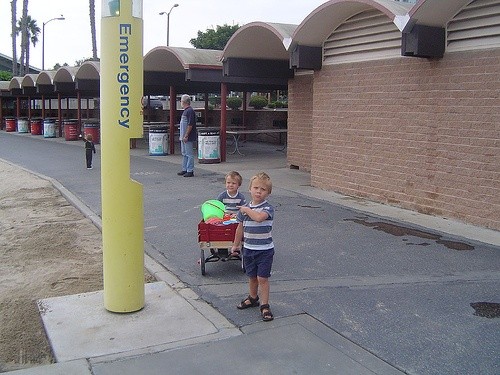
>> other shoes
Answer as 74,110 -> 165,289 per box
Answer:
87,167 -> 93,169
177,171 -> 187,175
184,172 -> 193,177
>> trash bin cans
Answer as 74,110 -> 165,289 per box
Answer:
148,126 -> 169,156
30,118 -> 41,135
43,118 -> 56,138
83,122 -> 99,144
63,120 -> 79,141
17,117 -> 28,133
197,127 -> 221,163
5,116 -> 15,131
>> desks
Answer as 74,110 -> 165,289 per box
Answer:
1,115 -> 288,156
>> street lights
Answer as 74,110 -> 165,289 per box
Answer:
159,4 -> 180,46
42,18 -> 65,70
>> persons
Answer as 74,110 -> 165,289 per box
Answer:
215,171 -> 247,256
79,134 -> 96,169
231,171 -> 275,321
177,94 -> 196,177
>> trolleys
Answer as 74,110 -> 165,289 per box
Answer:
197,207 -> 246,276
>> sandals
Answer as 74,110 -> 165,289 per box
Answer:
236,295 -> 260,309
260,304 -> 273,321
